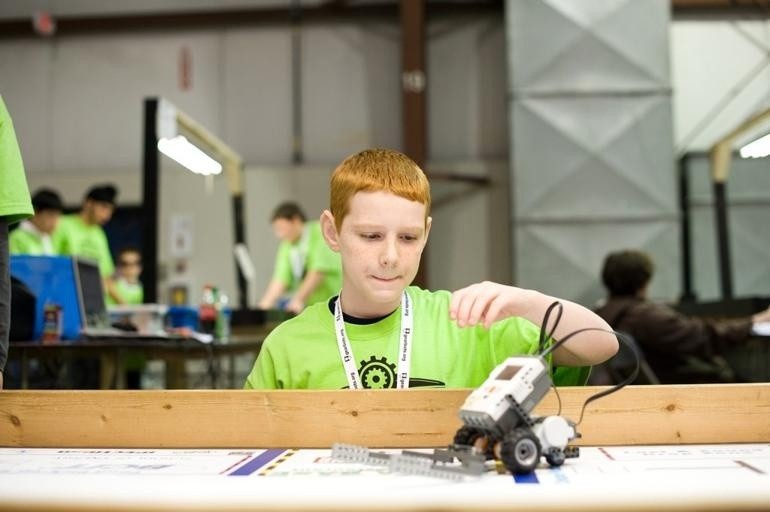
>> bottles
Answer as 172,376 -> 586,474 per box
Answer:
40,304 -> 63,342
200,285 -> 230,347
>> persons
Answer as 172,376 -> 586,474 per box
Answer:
238,144 -> 621,395
100,247 -> 150,388
37,179 -> 120,390
6,188 -> 64,257
0,95 -> 38,394
575,245 -> 770,382
254,200 -> 347,318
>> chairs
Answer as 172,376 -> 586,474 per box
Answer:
604,332 -> 662,384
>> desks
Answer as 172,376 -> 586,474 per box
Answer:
8,335 -> 266,390
112,310 -> 295,389
